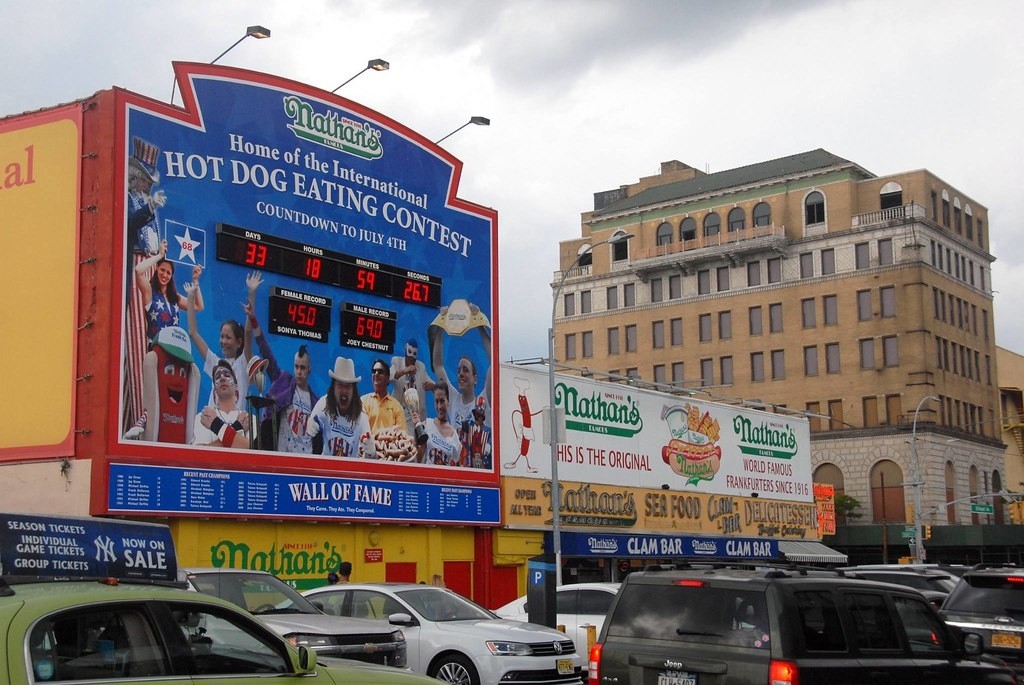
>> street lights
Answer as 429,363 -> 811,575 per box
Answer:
547,232 -> 638,587
911,396 -> 942,566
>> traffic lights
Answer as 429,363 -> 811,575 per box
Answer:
925,524 -> 932,541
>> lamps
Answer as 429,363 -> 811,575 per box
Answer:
732,200 -> 738,208
707,206 -> 713,214
370,58 -> 390,72
758,194 -> 765,203
751,491 -> 759,498
471,117 -> 491,126
662,483 -> 671,490
247,26 -> 271,39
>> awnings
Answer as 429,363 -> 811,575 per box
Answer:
777,541 -> 849,562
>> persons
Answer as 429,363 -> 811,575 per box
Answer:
122,138 -> 493,469
326,562 -> 361,616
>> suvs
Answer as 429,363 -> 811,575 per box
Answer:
587,559 -> 1021,685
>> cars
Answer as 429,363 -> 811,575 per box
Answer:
488,581 -> 757,680
1,511 -> 451,685
832,561 -> 1024,680
268,583 -> 585,685
177,566 -> 409,668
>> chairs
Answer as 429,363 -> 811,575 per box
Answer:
27,623 -> 48,650
94,626 -> 128,651
381,600 -> 393,615
354,603 -> 370,618
